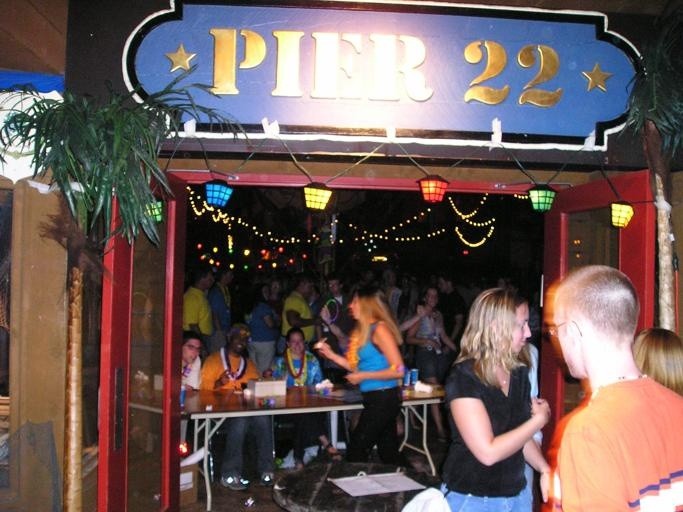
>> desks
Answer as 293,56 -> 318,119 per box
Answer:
128,381 -> 445,512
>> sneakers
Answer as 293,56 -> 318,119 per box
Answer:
220,470 -> 247,491
260,472 -> 275,486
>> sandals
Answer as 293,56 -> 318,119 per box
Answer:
325,443 -> 343,462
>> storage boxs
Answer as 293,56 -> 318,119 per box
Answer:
179,463 -> 199,507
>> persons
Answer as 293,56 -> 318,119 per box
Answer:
438,289 -> 552,511
632,328 -> 683,394
543,265 -> 683,512
181,255 -> 539,491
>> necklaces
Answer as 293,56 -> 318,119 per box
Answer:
587,370 -> 650,385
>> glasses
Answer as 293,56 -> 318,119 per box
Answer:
548,320 -> 582,336
